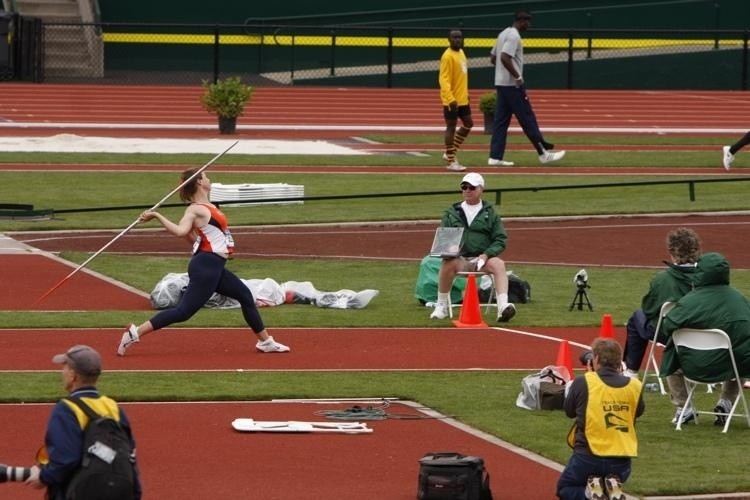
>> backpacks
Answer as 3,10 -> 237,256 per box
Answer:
66,395 -> 135,500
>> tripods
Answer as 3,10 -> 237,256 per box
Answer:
568,285 -> 594,312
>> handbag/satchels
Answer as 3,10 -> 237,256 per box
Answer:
522,368 -> 565,410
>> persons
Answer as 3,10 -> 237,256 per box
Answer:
117,167 -> 291,356
554,338 -> 646,500
26,344 -> 143,499
621,227 -> 703,367
429,172 -> 516,324
438,28 -> 474,171
722,130 -> 750,171
660,252 -> 750,427
486,10 -> 565,168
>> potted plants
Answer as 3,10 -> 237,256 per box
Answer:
200,74 -> 253,132
479,87 -> 499,134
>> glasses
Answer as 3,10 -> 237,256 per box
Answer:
460,186 -> 477,191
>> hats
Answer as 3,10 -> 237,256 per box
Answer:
52,344 -> 102,376
460,172 -> 485,189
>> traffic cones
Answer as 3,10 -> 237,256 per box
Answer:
600,315 -> 615,340
451,272 -> 492,333
596,313 -> 616,341
553,334 -> 576,382
557,340 -> 576,380
566,368 -> 576,375
453,272 -> 488,329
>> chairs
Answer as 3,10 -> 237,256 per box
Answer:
640,300 -> 714,395
672,329 -> 749,434
446,266 -> 498,318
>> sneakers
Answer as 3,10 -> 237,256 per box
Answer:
486,158 -> 514,167
584,475 -> 627,500
116,322 -> 139,356
714,399 -> 733,429
622,371 -> 641,381
255,336 -> 291,354
670,406 -> 698,426
722,145 -> 734,172
442,153 -> 468,171
430,301 -> 449,320
496,303 -> 514,324
538,149 -> 564,164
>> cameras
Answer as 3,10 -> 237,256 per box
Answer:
573,269 -> 588,286
580,351 -> 594,366
1,465 -> 47,483
